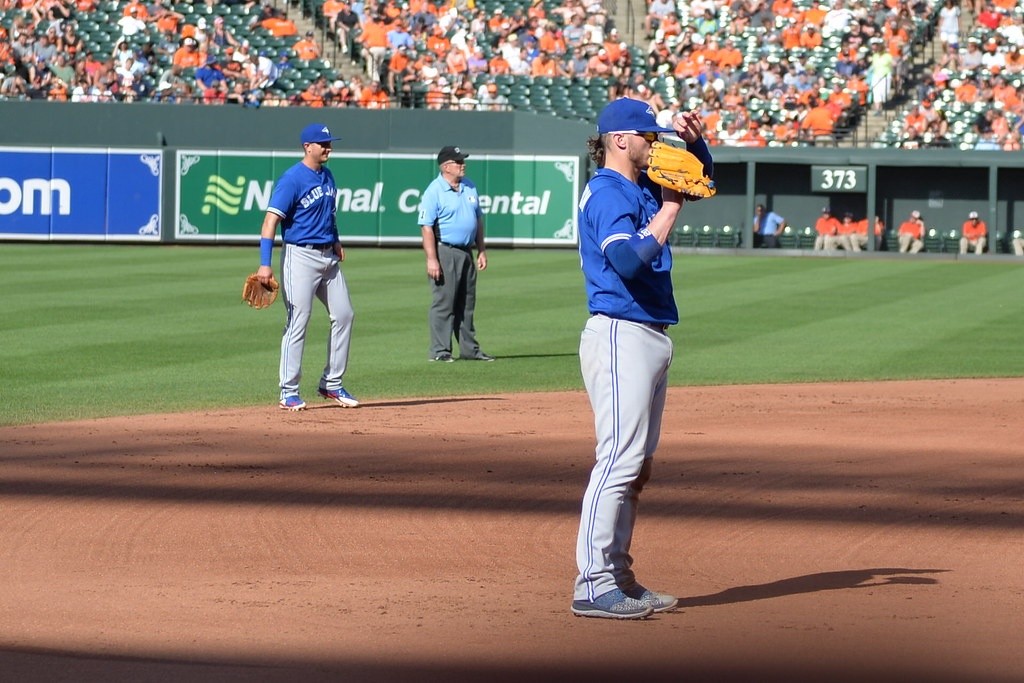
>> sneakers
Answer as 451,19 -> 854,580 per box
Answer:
474,352 -> 496,362
619,581 -> 680,613
428,351 -> 455,363
570,587 -> 654,620
279,395 -> 307,411
316,385 -> 360,408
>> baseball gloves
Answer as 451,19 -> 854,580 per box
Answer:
646,140 -> 718,199
240,273 -> 279,310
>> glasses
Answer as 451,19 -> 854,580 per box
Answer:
445,159 -> 465,165
605,130 -> 665,142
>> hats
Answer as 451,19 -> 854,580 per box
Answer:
437,144 -> 469,165
822,206 -> 831,214
911,210 -> 921,219
597,97 -> 678,133
969,211 -> 978,220
299,122 -> 342,145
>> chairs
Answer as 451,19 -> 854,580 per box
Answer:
672,225 -> 1024,255
0,0 -> 1024,151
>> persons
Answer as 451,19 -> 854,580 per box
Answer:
571,96 -> 714,618
752,204 -> 788,248
959,211 -> 986,255
257,121 -> 361,412
418,144 -> 494,362
812,206 -> 886,253
0,0 -> 1024,152
898,210 -> 925,254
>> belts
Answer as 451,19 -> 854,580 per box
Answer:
435,239 -> 471,250
616,319 -> 670,331
294,242 -> 334,249
